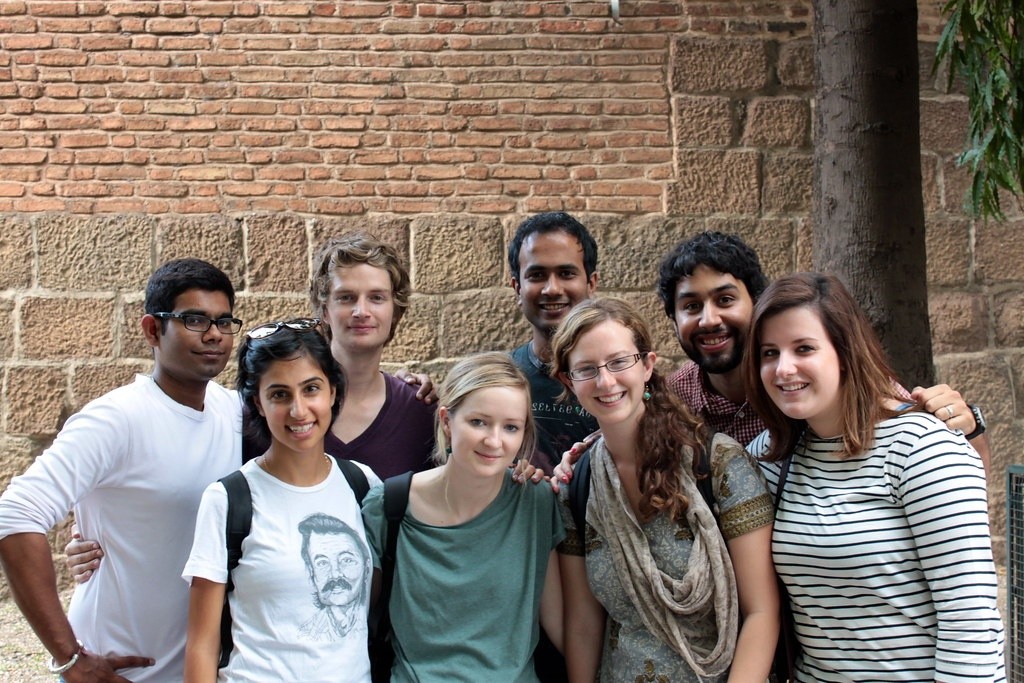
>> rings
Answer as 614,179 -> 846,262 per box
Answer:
946,406 -> 953,418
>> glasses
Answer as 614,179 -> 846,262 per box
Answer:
154,312 -> 243,334
567,352 -> 647,382
245,317 -> 322,339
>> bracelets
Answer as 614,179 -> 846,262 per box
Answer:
48,640 -> 84,674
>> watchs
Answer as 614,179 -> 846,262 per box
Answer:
965,404 -> 986,440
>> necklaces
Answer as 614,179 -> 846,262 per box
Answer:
263,454 -> 331,474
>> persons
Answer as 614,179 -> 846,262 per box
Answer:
181,328 -> 550,683
361,352 -> 566,683
65,240 -> 443,582
550,274 -> 1007,683
0,257 -> 440,683
658,235 -> 989,683
553,297 -> 782,683
504,211 -> 599,474
